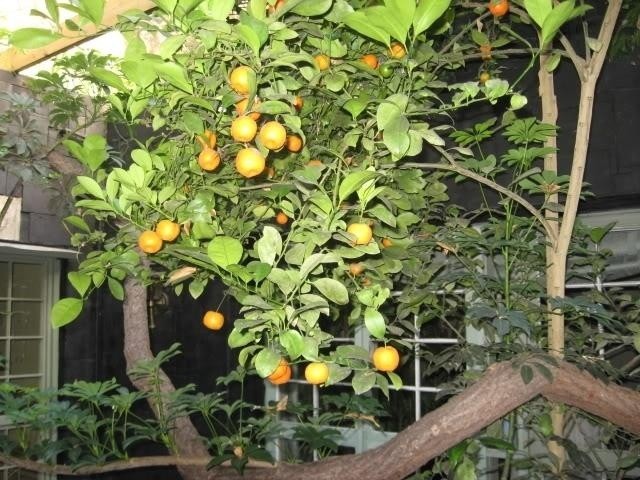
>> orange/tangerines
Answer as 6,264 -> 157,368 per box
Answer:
137,0 -> 508,387
